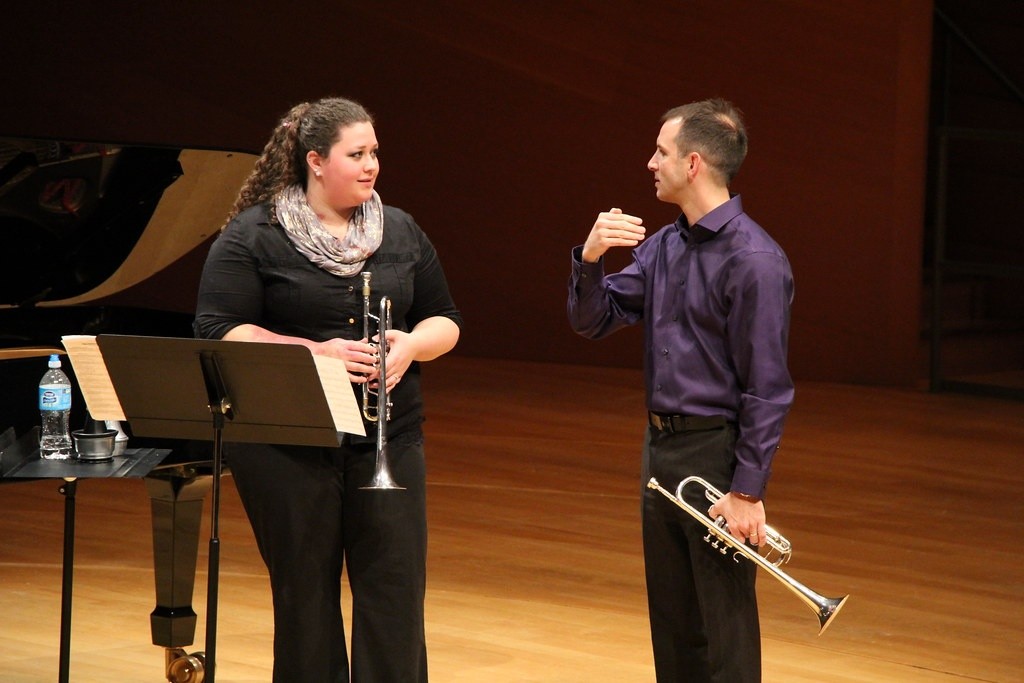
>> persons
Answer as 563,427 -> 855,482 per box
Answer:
566,100 -> 795,683
192,96 -> 462,682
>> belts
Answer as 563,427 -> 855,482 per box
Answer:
649,412 -> 728,434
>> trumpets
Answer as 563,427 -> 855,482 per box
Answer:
358,268 -> 409,492
646,473 -> 851,638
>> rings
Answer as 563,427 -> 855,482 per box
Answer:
750,531 -> 757,537
394,374 -> 399,379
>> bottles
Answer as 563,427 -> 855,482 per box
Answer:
39,355 -> 71,459
